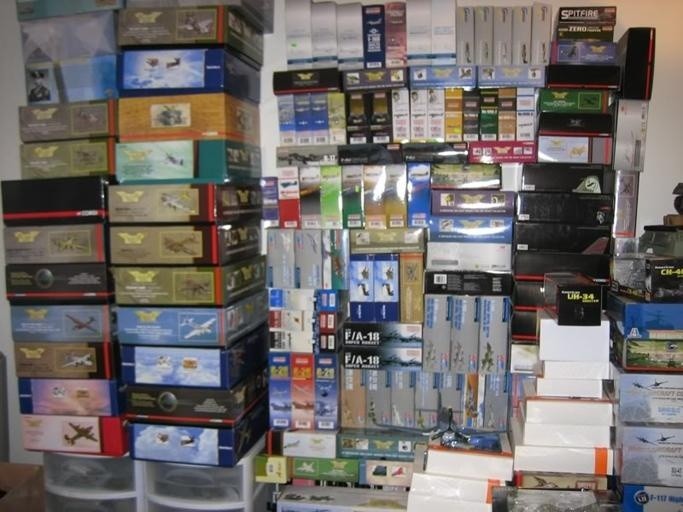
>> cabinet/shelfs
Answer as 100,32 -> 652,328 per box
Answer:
143,432 -> 270,512
42,451 -> 144,512
